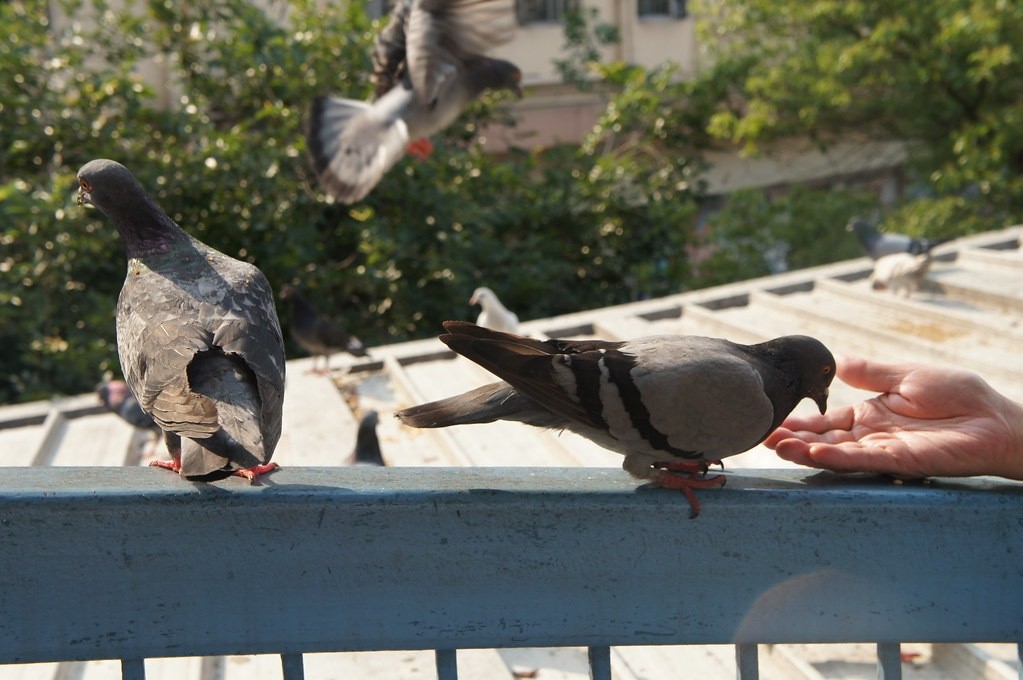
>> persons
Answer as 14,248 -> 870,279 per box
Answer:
761,354 -> 1022,486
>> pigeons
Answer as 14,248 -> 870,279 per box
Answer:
78,159 -> 286,480
846,218 -> 960,298
279,286 -> 835,518
304,2 -> 523,204
